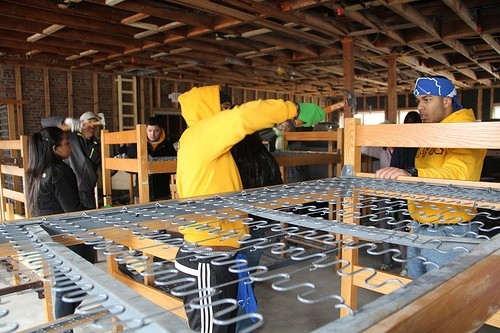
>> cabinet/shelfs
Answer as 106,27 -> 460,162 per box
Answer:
0,118 -> 500,332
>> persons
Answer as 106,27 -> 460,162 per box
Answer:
26,126 -> 93,333
174,84 -> 325,333
41,112 -> 177,263
230,76 -> 488,289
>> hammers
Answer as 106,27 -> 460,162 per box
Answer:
292,88 -> 357,127
60,112 -> 106,133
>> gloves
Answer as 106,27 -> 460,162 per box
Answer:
65,118 -> 81,132
294,101 -> 326,127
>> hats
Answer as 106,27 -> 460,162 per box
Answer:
79,111 -> 100,122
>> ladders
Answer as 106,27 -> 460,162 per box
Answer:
118,74 -> 138,148
